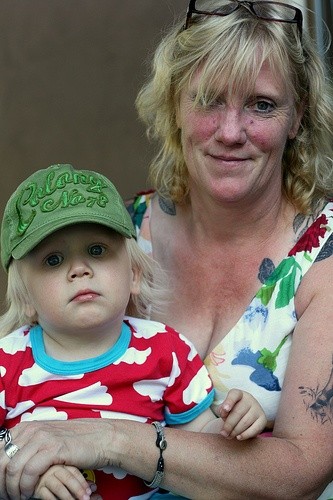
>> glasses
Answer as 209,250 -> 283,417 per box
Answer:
185,0 -> 303,45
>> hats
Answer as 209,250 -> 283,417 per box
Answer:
1,162 -> 138,274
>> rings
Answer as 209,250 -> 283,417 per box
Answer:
0,428 -> 12,444
4,442 -> 20,458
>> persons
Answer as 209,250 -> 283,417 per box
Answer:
0,0 -> 333,500
143,422 -> 167,488
0,163 -> 267,500
